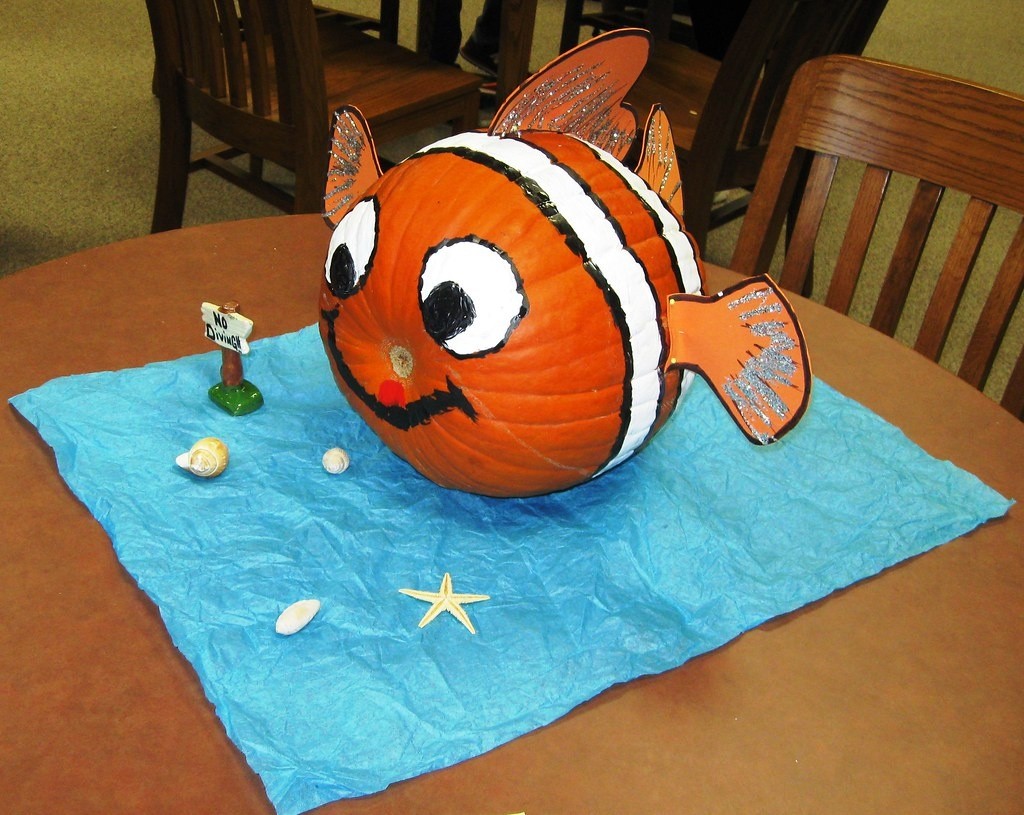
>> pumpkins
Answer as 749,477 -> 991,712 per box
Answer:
317,131 -> 709,501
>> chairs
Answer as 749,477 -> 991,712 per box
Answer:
143,0 -> 483,237
729,55 -> 1024,421
556,0 -> 888,296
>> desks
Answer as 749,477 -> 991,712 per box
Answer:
0,215 -> 1023,815
